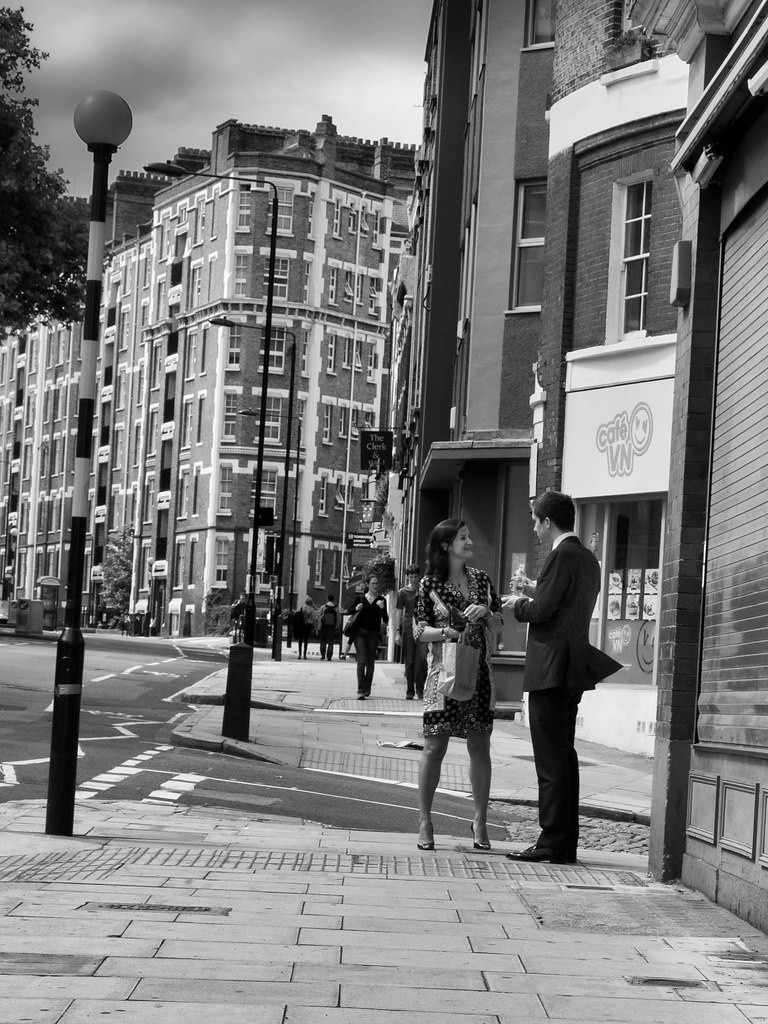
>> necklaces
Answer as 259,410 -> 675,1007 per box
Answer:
451,576 -> 461,589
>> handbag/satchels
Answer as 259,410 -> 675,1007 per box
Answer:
230,600 -> 242,619
343,594 -> 363,637
437,631 -> 480,701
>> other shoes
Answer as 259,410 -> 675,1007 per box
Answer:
321,655 -> 325,659
340,656 -> 346,660
416,690 -> 424,698
406,693 -> 414,699
365,688 -> 371,696
357,691 -> 365,700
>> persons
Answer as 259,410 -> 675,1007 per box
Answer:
414,519 -> 504,852
298,595 -> 341,661
501,492 -> 623,863
348,574 -> 389,700
395,565 -> 429,700
231,592 -> 247,619
340,638 -> 353,660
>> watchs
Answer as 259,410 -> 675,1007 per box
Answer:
442,628 -> 450,639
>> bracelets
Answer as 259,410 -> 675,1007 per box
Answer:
395,629 -> 400,632
482,604 -> 489,617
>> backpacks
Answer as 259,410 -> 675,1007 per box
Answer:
322,605 -> 337,627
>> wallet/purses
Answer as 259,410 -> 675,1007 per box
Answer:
448,605 -> 467,632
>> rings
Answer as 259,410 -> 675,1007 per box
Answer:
475,611 -> 478,613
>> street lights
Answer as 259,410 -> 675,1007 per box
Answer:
43,90 -> 133,835
141,159 -> 277,650
208,315 -> 296,661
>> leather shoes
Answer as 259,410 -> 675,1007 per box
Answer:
506,842 -> 578,865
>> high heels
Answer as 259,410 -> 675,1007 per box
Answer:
471,823 -> 491,850
417,820 -> 434,849
